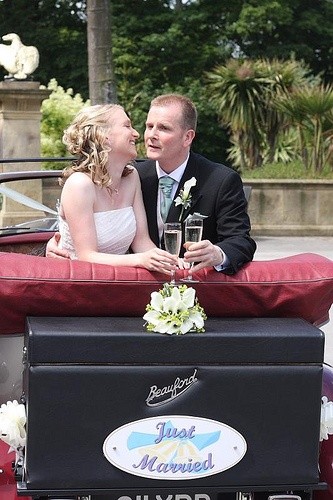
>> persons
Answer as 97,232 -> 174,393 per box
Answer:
55,104 -> 181,276
44,94 -> 258,277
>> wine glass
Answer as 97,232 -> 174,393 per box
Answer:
163,222 -> 181,287
179,218 -> 203,283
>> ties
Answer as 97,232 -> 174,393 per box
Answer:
159,177 -> 174,224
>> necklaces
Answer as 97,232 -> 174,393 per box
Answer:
109,183 -> 119,195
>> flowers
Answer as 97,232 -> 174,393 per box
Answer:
173,176 -> 196,222
142,283 -> 207,335
